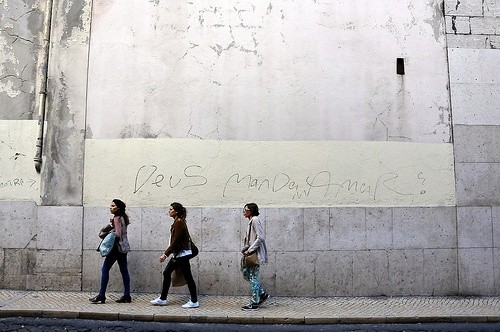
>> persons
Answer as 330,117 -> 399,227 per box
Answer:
239,203 -> 270,311
88,199 -> 131,303
149,202 -> 200,308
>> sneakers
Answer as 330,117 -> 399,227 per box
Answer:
258,293 -> 270,307
182,299 -> 199,308
150,296 -> 167,305
241,304 -> 259,310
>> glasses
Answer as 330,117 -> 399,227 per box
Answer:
243,208 -> 250,211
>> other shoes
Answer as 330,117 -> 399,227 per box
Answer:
115,296 -> 132,303
89,295 -> 106,304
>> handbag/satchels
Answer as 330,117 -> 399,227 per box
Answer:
189,241 -> 198,260
117,241 -> 130,253
99,230 -> 116,257
243,251 -> 258,266
170,270 -> 187,287
